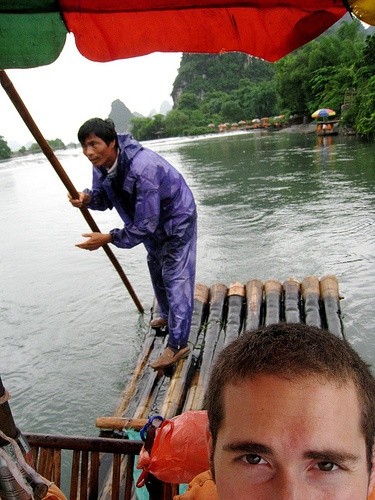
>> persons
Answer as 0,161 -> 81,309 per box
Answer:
206,323 -> 375,500
67,118 -> 197,369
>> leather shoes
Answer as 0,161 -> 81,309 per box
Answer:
150,317 -> 167,327
150,345 -> 190,369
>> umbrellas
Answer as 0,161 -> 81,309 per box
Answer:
311,109 -> 336,117
0,0 -> 374,70
209,115 -> 285,127
